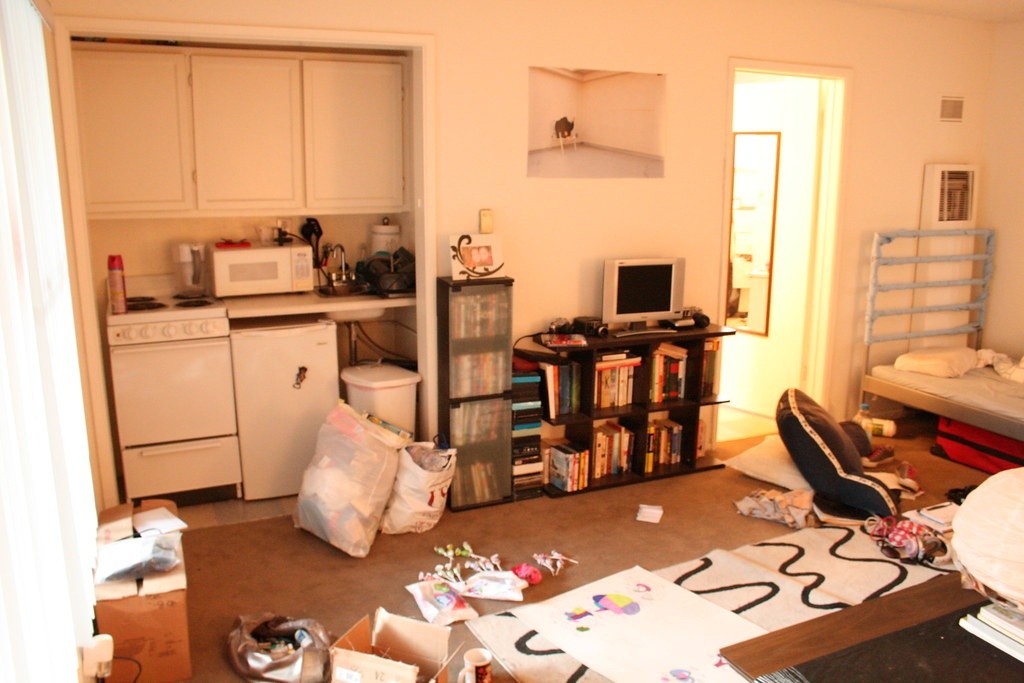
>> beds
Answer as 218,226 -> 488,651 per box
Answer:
859,227 -> 1024,464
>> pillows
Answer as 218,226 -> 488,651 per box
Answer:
895,346 -> 981,378
724,432 -> 816,491
776,388 -> 901,518
836,421 -> 872,457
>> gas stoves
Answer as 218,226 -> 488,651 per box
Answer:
105,273 -> 230,346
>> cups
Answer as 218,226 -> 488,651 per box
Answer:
458,648 -> 492,683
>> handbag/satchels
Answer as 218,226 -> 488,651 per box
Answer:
377,442 -> 457,533
292,399 -> 410,558
226,611 -> 333,683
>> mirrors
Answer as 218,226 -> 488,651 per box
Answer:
719,131 -> 782,334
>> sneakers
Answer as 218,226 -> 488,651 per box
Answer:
894,461 -> 920,492
861,444 -> 895,467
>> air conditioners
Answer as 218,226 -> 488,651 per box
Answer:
911,161 -> 981,349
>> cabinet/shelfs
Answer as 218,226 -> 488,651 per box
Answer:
513,320 -> 738,496
71,40 -> 411,221
437,274 -> 514,511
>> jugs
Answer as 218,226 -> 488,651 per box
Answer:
172,243 -> 205,299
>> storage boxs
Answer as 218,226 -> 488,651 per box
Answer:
93,499 -> 191,683
331,606 -> 467,683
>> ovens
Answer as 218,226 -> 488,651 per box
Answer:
109,337 -> 243,501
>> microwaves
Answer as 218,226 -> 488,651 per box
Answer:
209,238 -> 314,298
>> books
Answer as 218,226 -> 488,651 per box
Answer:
543,405 -> 720,493
538,338 -> 720,418
452,458 -> 500,506
451,352 -> 507,397
509,372 -> 544,491
452,291 -> 511,338
452,400 -> 510,444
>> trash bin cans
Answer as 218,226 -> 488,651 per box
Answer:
340,363 -> 421,442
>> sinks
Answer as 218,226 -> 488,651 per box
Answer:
313,285 -> 375,298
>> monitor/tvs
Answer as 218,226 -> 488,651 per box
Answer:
601,258 -> 685,338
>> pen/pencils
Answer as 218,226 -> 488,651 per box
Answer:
928,503 -> 952,510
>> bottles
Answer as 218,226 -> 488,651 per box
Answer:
371,218 -> 400,256
259,630 -> 313,660
858,405 -> 872,444
853,416 -> 896,437
108,255 -> 127,314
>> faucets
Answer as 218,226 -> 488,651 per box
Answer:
332,244 -> 348,282
